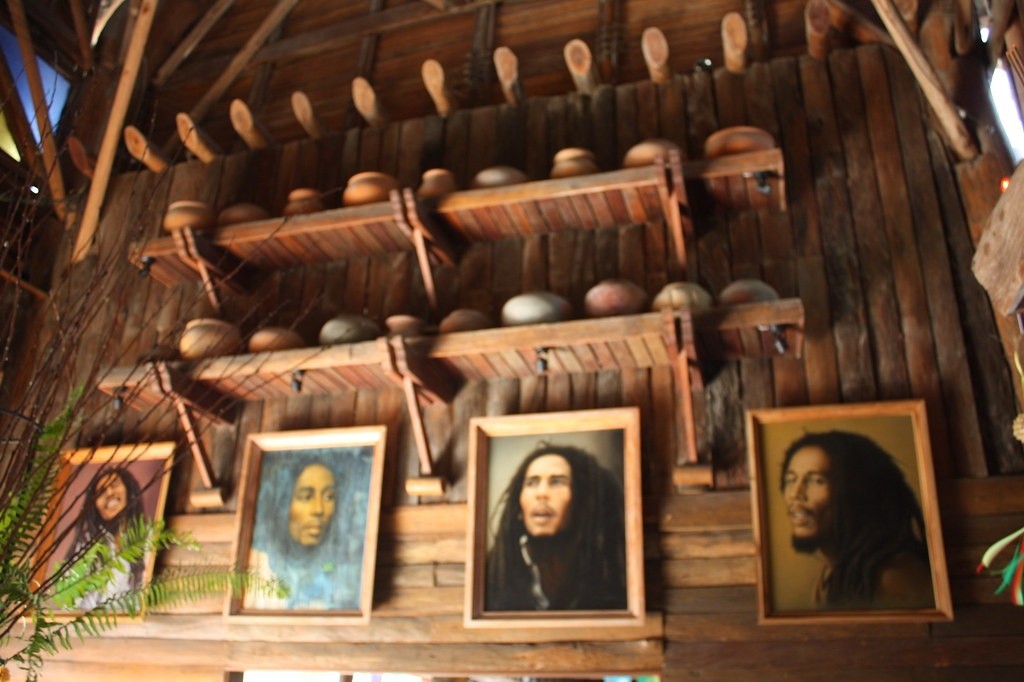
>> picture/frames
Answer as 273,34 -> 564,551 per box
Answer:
744,400 -> 953,630
20,441 -> 175,625
462,405 -> 648,634
220,424 -> 390,627
